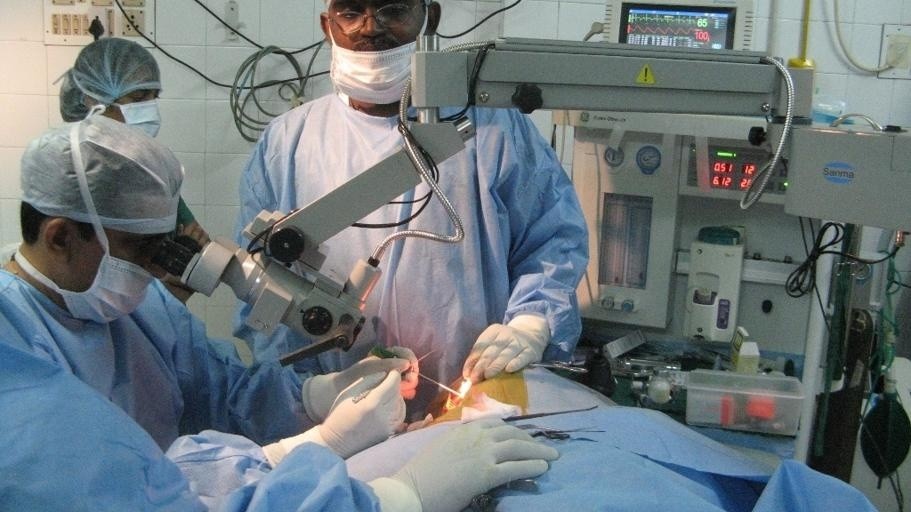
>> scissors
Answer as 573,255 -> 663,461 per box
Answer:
527,359 -> 588,374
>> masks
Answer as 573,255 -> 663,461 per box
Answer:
56,243 -> 157,324
118,95 -> 164,138
328,39 -> 419,104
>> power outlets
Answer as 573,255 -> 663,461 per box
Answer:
875,21 -> 911,79
43,0 -> 156,49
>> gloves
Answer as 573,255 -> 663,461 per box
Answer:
263,374 -> 407,471
463,309 -> 551,384
302,347 -> 419,424
368,417 -> 559,512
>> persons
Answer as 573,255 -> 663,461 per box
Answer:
2,105 -> 561,512
53,39 -> 216,305
235,3 -> 591,385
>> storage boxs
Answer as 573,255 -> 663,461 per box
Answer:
685,367 -> 807,436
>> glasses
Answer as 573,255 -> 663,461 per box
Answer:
327,2 -> 419,36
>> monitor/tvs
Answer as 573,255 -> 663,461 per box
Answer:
602,0 -> 757,53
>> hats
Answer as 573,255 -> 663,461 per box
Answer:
18,117 -> 186,235
